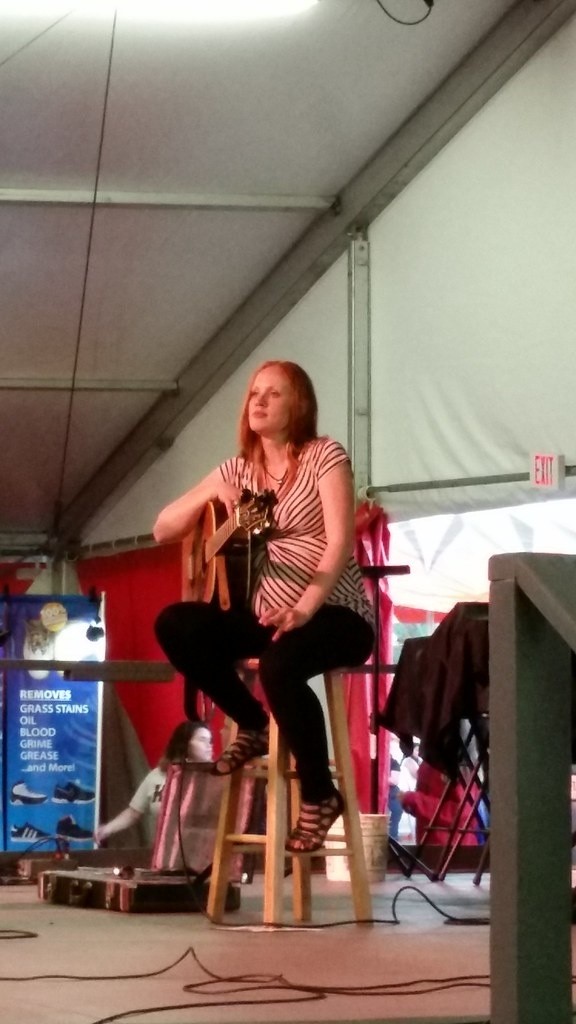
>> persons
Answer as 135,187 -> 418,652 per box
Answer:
152,361 -> 376,852
389,716 -> 488,845
94,722 -> 213,849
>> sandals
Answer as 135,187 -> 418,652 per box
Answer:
285,789 -> 344,853
210,713 -> 271,778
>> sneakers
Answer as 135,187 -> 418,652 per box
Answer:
11,823 -> 52,842
10,780 -> 48,805
52,783 -> 96,804
55,815 -> 93,842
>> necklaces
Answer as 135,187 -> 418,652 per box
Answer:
265,469 -> 288,484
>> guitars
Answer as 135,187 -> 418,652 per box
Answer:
180,494 -> 278,605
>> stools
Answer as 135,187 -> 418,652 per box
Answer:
206,658 -> 377,927
